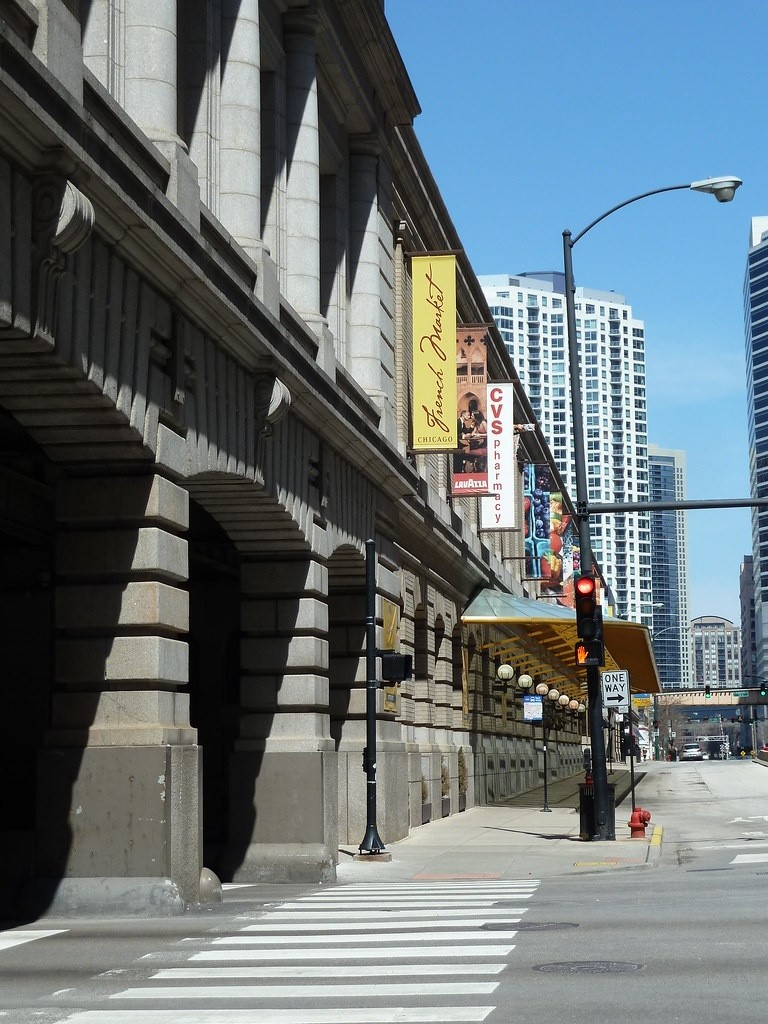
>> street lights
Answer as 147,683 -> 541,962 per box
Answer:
558,173 -> 747,841
499,663 -> 588,812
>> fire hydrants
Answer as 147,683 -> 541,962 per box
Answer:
627,806 -> 653,841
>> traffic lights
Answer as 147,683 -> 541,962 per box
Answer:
760,682 -> 767,696
573,574 -> 597,639
705,685 -> 711,699
575,641 -> 603,666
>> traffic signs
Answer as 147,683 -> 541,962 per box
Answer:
602,670 -> 631,708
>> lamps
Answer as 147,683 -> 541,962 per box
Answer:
546,689 -> 559,710
513,674 -> 534,701
491,664 -> 515,694
566,700 -> 579,718
573,704 -> 585,720
552,694 -> 570,714
528,683 -> 549,699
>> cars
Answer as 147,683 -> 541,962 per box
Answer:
678,743 -> 703,760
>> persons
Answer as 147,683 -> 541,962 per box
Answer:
643,747 -> 647,761
464,410 -> 487,473
454,410 -> 475,473
670,746 -> 677,762
